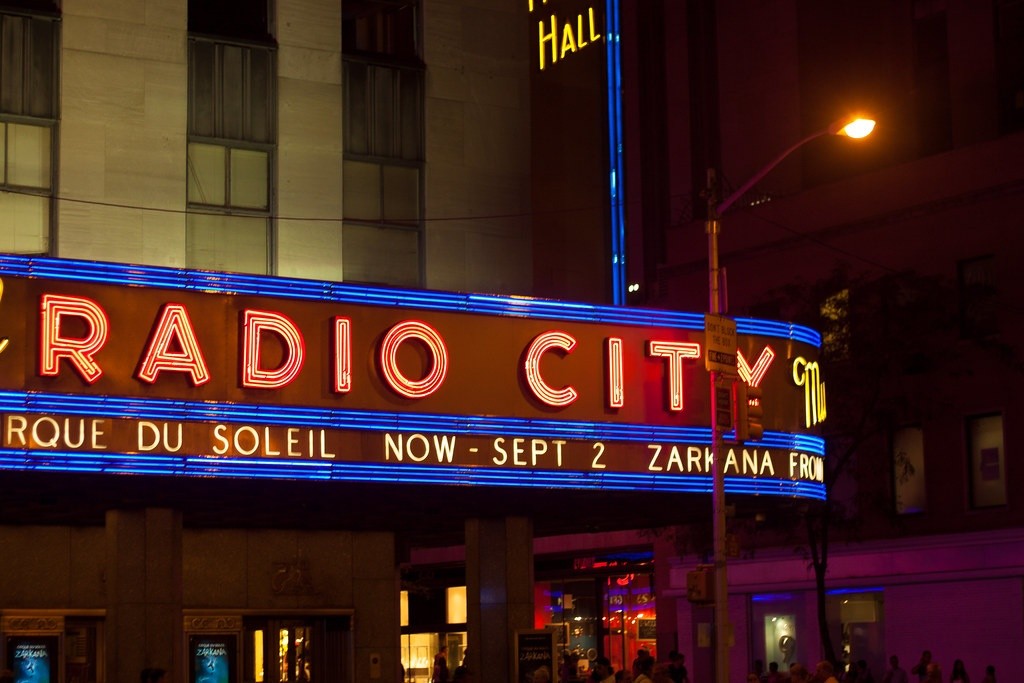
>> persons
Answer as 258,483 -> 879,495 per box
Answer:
533,651 -> 690,683
748,650 -> 996,683
432,646 -> 471,683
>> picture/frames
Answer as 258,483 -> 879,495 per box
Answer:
547,622 -> 570,647
636,618 -> 657,641
513,628 -> 559,683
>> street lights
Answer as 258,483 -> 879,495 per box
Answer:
704,111 -> 878,683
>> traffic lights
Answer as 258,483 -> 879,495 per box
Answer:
733,379 -> 764,443
